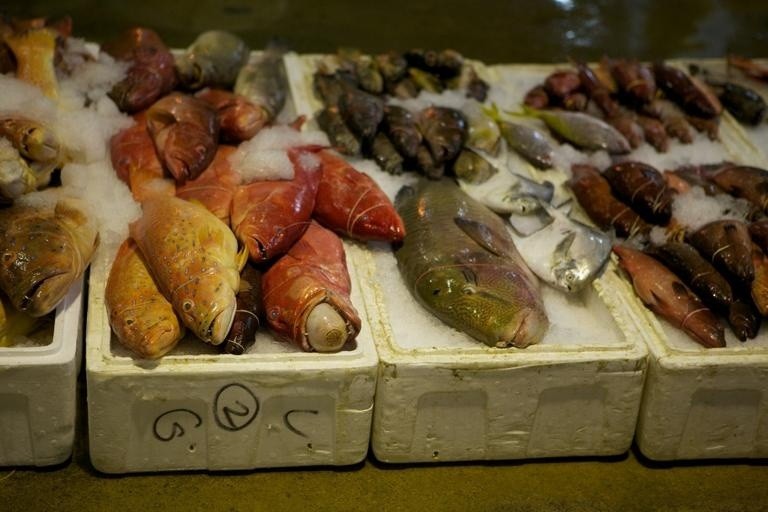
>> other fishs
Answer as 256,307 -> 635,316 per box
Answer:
0,13 -> 768,361
